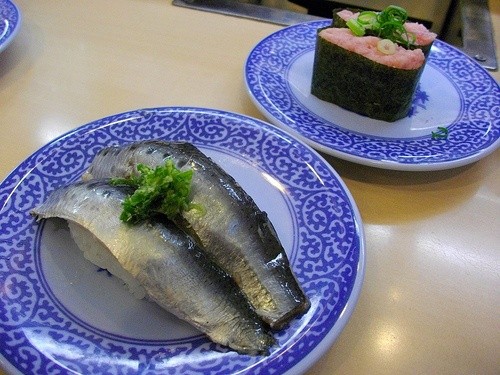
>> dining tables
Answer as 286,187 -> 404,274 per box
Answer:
1,0 -> 500,375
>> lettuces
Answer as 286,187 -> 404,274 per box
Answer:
107,158 -> 205,226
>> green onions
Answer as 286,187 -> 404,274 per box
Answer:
347,4 -> 415,54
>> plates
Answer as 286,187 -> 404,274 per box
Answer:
243,19 -> 500,171
0,106 -> 365,375
0,0 -> 22,54
